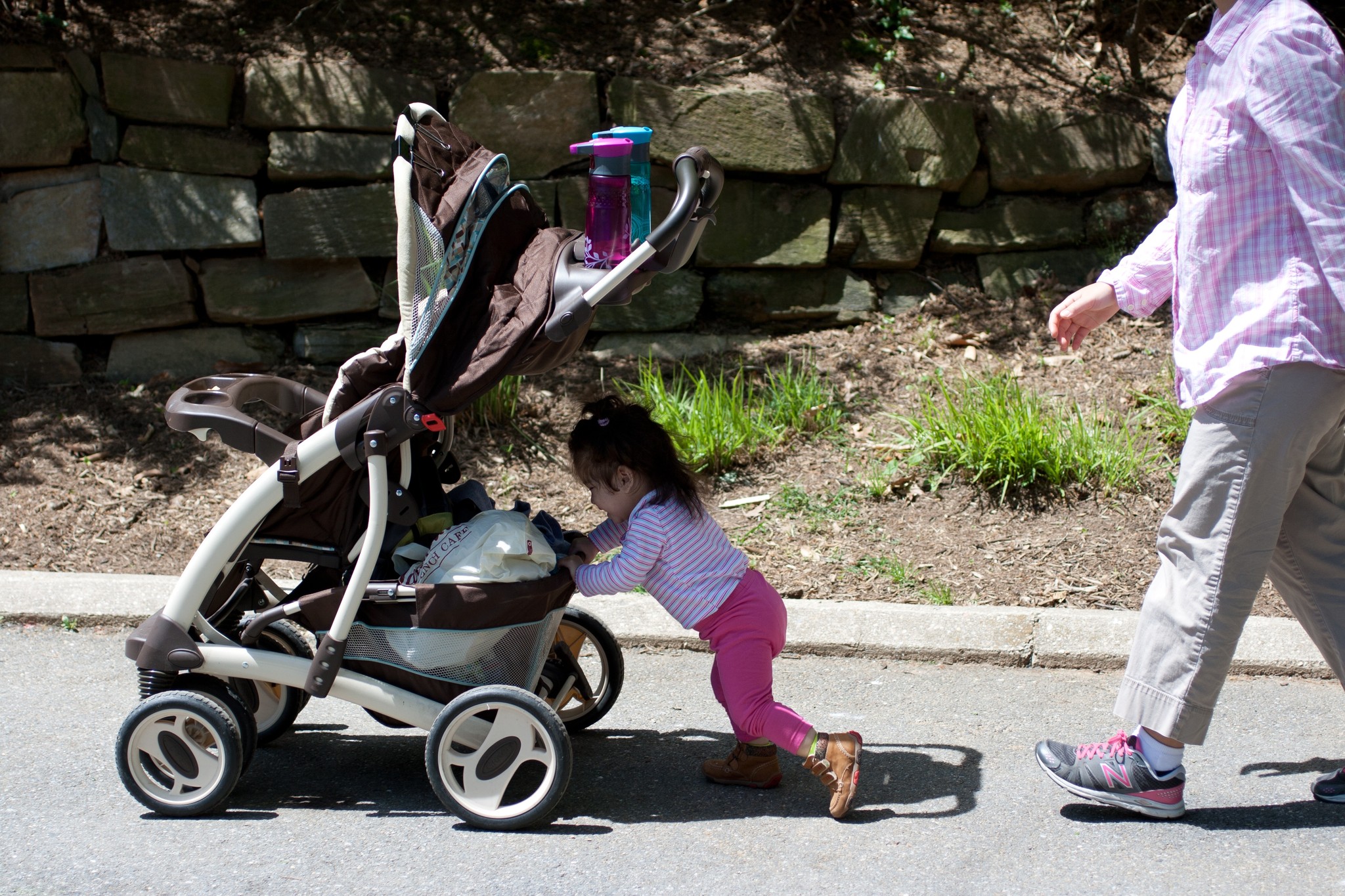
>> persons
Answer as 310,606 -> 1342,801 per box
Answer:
553,393 -> 863,821
1031,0 -> 1345,819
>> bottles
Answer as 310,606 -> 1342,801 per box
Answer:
592,125 -> 653,252
568,138 -> 633,267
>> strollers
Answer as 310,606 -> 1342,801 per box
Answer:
113,100 -> 725,831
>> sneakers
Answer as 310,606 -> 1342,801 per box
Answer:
1311,767 -> 1345,808
701,741 -> 783,790
1034,735 -> 1189,820
801,731 -> 864,822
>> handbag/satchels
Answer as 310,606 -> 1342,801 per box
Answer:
395,504 -> 557,589
